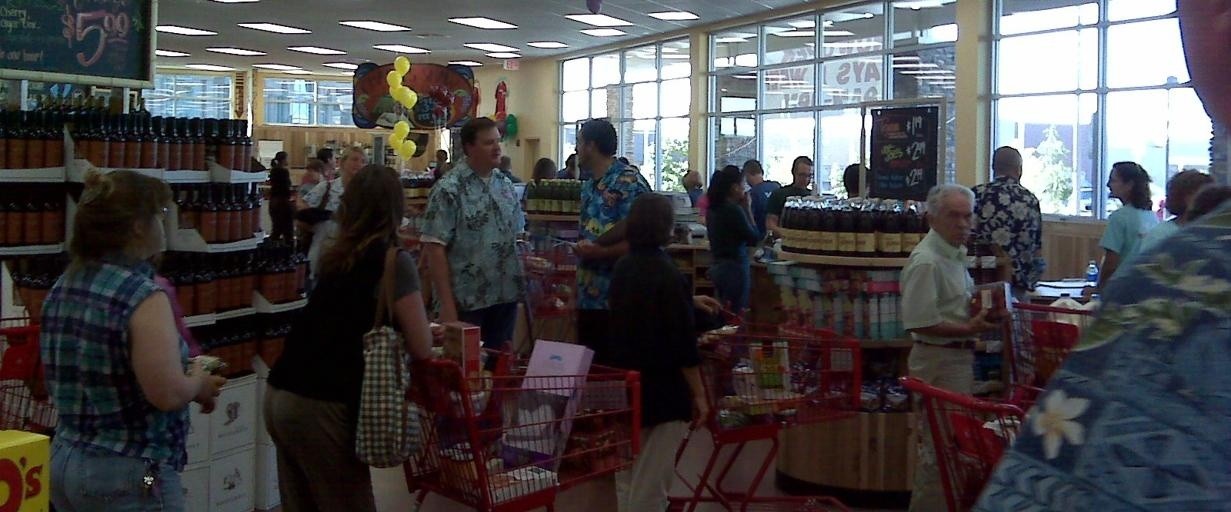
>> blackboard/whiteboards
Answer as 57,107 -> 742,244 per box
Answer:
867,105 -> 939,202
0,0 -> 159,88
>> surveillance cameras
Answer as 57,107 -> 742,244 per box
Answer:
584,0 -> 602,16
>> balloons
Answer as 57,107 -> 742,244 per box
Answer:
386,56 -> 418,161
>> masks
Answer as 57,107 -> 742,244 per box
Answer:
133,215 -> 169,260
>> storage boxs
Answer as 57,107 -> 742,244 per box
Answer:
180,356 -> 282,512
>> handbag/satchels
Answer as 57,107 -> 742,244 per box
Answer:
353,320 -> 423,470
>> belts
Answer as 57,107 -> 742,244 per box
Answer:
914,338 -> 978,352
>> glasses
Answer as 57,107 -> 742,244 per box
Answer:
794,171 -> 814,178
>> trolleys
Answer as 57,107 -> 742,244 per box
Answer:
1005,302 -> 1096,387
897,375 -> 1046,511
517,244 -> 583,351
1,307 -> 58,446
396,346 -> 643,511
667,326 -> 865,512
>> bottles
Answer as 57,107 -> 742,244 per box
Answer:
1,103 -> 252,171
184,314 -> 293,375
399,170 -> 434,198
1085,259 -> 1100,298
177,179 -> 266,242
525,179 -> 585,215
159,237 -> 313,307
777,191 -> 929,258
773,328 -> 790,371
10,260 -> 62,321
0,183 -> 62,248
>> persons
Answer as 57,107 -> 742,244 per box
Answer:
575,119 -> 652,366
1098,160 -> 1160,288
623,200 -> 735,511
897,183 -> 1003,509
684,155 -> 814,314
264,117 -> 575,511
1140,169 -> 1215,252
971,145 -> 1046,400
1185,185 -> 1229,219
40,164 -> 226,512
843,164 -> 871,198
975,0 -> 1231,512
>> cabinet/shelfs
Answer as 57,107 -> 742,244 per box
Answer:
0,124 -> 312,329
